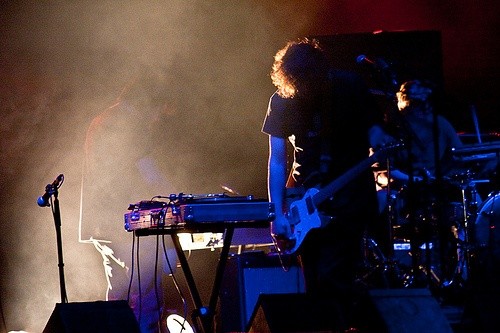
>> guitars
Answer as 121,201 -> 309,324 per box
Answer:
274,138 -> 408,254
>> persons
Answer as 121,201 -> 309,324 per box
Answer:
379,75 -> 465,275
262,39 -> 412,302
79,71 -> 174,333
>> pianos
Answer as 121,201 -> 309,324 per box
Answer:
124,195 -> 280,230
175,228 -> 279,252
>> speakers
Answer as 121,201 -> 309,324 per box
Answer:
219,252 -> 305,333
42,300 -> 141,333
245,288 -> 452,333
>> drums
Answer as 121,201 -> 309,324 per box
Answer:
389,180 -> 500,288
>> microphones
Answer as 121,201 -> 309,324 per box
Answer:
37,174 -> 63,207
357,56 -> 385,71
